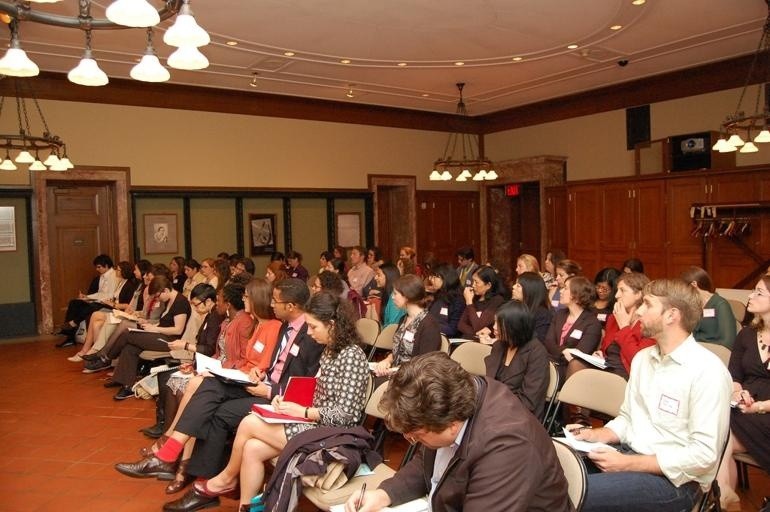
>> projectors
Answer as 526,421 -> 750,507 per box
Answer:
681,138 -> 704,152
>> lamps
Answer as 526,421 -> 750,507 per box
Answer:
0,1 -> 213,90
425,83 -> 501,183
1,74 -> 74,174
713,3 -> 770,156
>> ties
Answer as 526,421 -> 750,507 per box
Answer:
264,325 -> 293,375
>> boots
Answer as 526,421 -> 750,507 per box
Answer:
143,402 -> 166,439
139,434 -> 169,459
164,458 -> 190,495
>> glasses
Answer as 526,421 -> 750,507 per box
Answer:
272,296 -> 297,308
596,287 -> 613,294
200,266 -> 210,269
193,298 -> 209,307
751,289 -> 770,297
154,292 -> 162,299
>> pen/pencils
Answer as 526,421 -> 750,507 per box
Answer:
255,369 -> 259,378
279,384 -> 283,397
356,482 -> 366,512
157,337 -> 170,344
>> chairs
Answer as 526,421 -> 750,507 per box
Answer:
140,309 -> 770,512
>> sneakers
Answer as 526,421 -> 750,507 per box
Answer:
112,388 -> 137,400
66,352 -> 86,363
104,380 -> 123,389
78,353 -> 112,375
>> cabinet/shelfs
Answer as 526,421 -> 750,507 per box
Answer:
760,164 -> 770,255
667,255 -> 760,289
567,181 -> 602,255
545,186 -> 566,257
602,255 -> 667,281
568,255 -> 602,283
602,174 -> 667,254
667,164 -> 760,255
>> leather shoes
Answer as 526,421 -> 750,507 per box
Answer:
192,478 -> 239,501
113,454 -> 178,483
54,338 -> 77,349
161,488 -> 223,512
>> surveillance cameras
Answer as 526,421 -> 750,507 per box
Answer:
617,58 -> 629,66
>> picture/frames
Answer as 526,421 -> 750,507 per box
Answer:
247,212 -> 278,257
141,212 -> 180,257
335,211 -> 363,250
